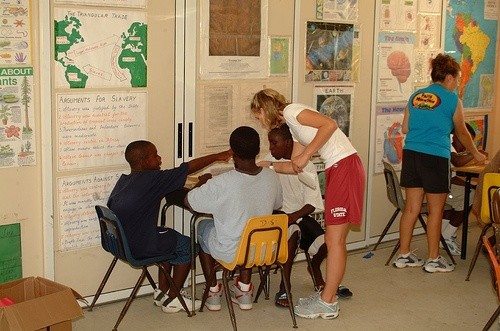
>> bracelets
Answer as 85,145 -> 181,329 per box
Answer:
269,162 -> 273,169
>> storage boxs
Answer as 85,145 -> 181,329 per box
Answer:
0,276 -> 89,331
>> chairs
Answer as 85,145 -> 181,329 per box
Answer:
86,205 -> 192,331
372,159 -> 457,266
199,214 -> 298,331
465,173 -> 500,331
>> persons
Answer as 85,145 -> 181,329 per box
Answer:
251,89 -> 366,318
391,55 -> 487,272
440,123 -> 488,255
107,141 -> 234,313
185,127 -> 284,310
256,124 -> 353,308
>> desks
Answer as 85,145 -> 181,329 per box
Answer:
161,187 -> 290,316
449,158 -> 491,260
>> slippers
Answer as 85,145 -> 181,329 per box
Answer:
335,284 -> 352,298
275,292 -> 289,307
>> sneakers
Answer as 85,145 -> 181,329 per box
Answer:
393,247 -> 425,268
201,283 -> 224,311
153,289 -> 202,312
423,255 -> 454,272
230,277 -> 254,309
440,235 -> 462,255
294,286 -> 340,318
